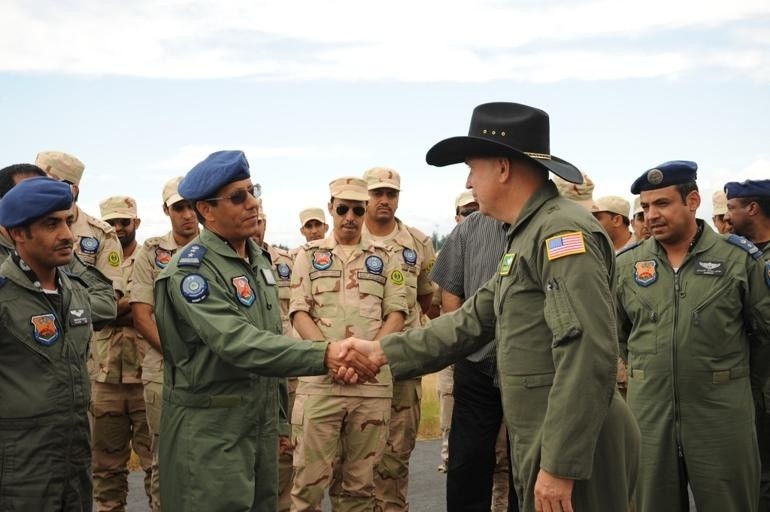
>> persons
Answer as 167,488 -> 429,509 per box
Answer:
251,196 -> 297,512
610,161 -> 770,511
152,149 -> 382,512
0,164 -> 119,332
84,196 -> 152,512
0,177 -> 96,512
332,102 -> 644,512
33,149 -> 127,378
126,178 -> 203,512
426,168 -> 652,512
360,167 -> 437,512
298,208 -> 330,242
288,177 -> 412,512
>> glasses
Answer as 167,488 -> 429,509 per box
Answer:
456,209 -> 473,216
203,184 -> 261,204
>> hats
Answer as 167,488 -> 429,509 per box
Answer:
162,149 -> 251,207
712,179 -> 770,217
455,191 -> 475,207
426,104 -> 583,185
0,150 -> 138,227
552,160 -> 698,218
300,168 -> 401,228
